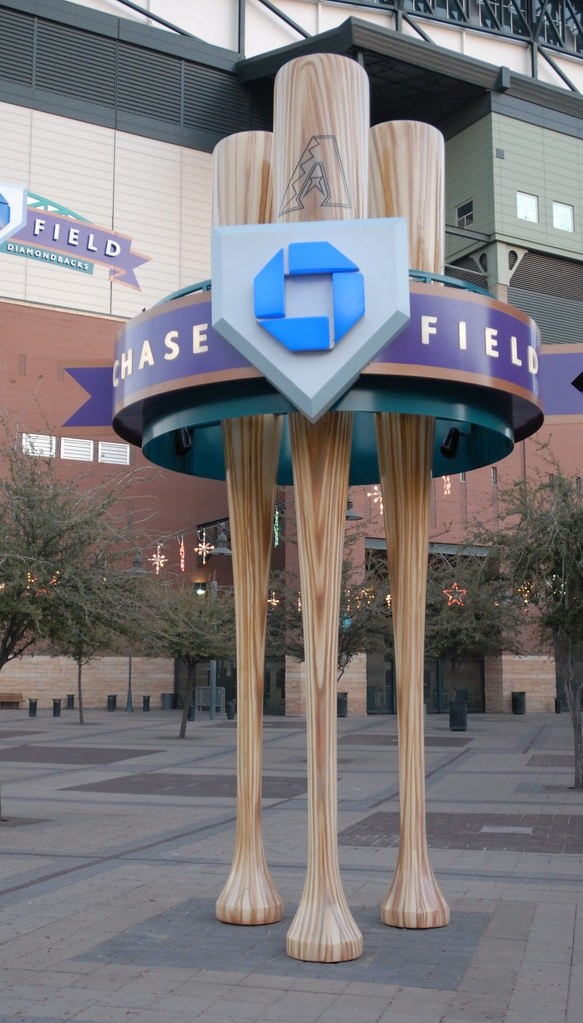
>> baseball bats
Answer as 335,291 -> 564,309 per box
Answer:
269,51 -> 372,967
365,119 -> 451,930
210,129 -> 286,928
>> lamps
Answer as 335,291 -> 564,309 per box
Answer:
438,427 -> 464,459
173,427 -> 192,454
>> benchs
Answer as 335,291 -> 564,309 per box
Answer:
0,693 -> 25,710
